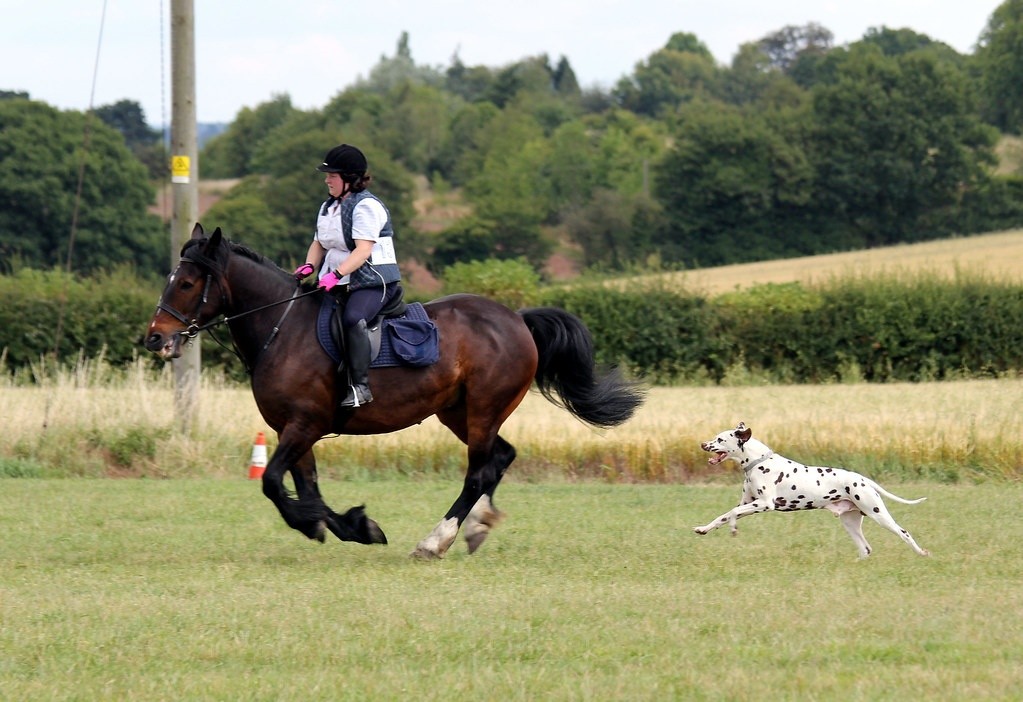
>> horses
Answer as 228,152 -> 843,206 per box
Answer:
143,220 -> 646,567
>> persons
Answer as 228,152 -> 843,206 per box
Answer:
287,144 -> 401,406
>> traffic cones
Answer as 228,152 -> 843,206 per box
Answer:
248,432 -> 267,480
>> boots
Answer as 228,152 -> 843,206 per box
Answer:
340,318 -> 374,406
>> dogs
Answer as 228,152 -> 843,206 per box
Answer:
693,421 -> 930,563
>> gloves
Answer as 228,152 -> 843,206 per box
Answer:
293,263 -> 315,280
317,269 -> 343,292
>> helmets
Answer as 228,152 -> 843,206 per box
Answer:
316,144 -> 367,173
